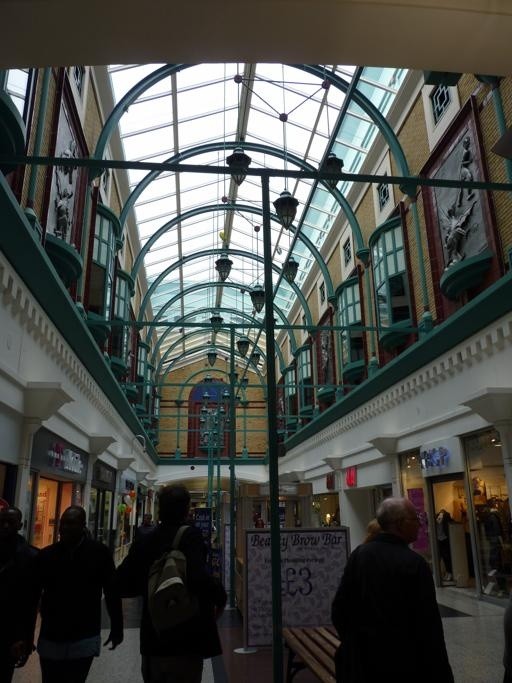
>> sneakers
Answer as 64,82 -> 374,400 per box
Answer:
442,573 -> 452,580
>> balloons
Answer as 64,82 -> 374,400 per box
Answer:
118,490 -> 136,513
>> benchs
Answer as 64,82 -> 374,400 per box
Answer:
280,622 -> 351,681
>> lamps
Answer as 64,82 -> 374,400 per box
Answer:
196,197 -> 269,417
225,75 -> 252,185
273,109 -> 300,285
320,80 -> 345,189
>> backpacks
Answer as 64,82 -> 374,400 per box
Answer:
149,525 -> 192,640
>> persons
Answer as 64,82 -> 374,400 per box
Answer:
11,506 -> 123,683
331,497 -> 454,683
0,506 -> 40,683
485,513 -> 506,597
364,518 -> 382,542
117,484 -> 226,683
136,514 -> 155,539
435,509 -> 452,581
